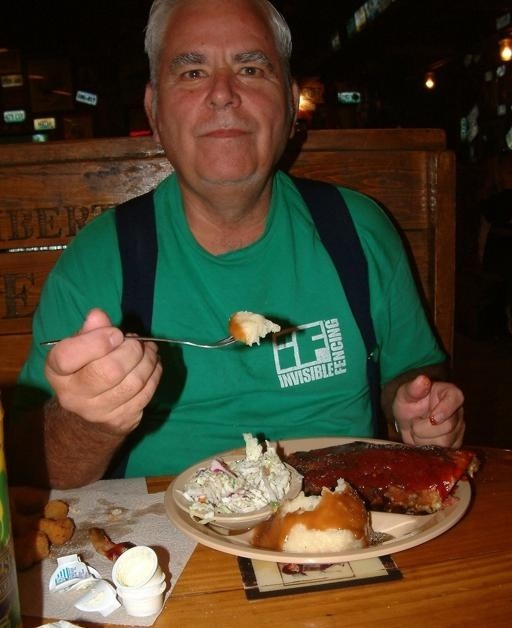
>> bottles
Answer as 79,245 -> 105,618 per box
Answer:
0,407 -> 23,627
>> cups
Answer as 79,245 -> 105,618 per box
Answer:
111,543 -> 168,618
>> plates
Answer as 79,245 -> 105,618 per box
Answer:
162,436 -> 470,566
172,456 -> 304,531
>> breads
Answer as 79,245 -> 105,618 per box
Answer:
230,311 -> 280,347
252,478 -> 369,554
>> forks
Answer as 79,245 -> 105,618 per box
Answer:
41,334 -> 236,349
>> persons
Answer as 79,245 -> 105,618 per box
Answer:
8,1 -> 469,489
474,144 -> 512,345
